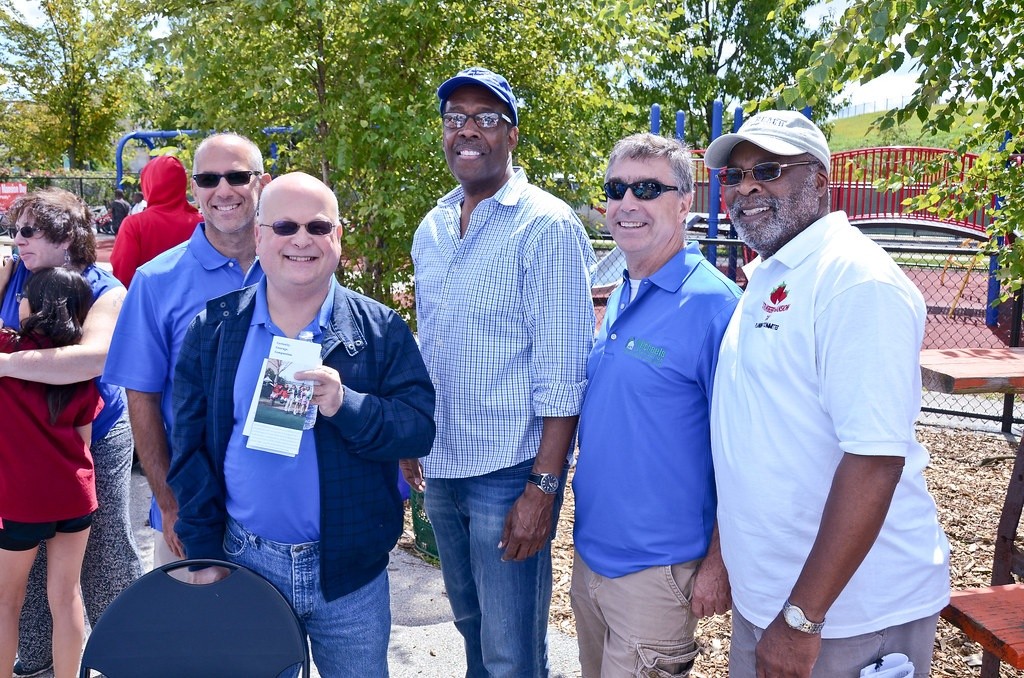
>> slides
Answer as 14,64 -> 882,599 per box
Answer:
593,213 -> 702,285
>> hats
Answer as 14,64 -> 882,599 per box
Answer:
704,110 -> 831,173
438,67 -> 518,125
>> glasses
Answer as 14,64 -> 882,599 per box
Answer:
7,226 -> 50,238
442,112 -> 512,129
604,180 -> 678,200
15,292 -> 29,303
259,221 -> 336,235
193,170 -> 262,189
714,160 -> 818,186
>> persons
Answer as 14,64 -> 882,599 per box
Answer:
572,130 -> 741,678
101,133 -> 271,583
169,172 -> 435,678
267,383 -> 312,417
387,64 -> 596,678
702,108 -> 951,678
0,154 -> 205,678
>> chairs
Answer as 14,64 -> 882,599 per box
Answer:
80,558 -> 310,678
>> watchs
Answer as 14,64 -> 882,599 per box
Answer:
781,598 -> 825,635
528,471 -> 562,495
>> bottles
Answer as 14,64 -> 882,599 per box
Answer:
290,332 -> 321,429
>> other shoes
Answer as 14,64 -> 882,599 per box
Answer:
13,658 -> 54,677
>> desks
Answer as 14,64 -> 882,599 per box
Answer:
919,346 -> 1024,678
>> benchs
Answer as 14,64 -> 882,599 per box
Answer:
938,582 -> 1023,671
